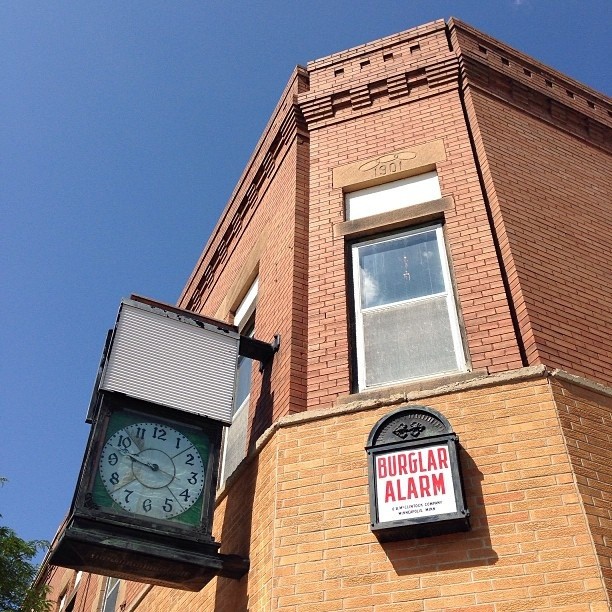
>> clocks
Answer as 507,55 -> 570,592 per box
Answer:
75,388 -> 224,545
